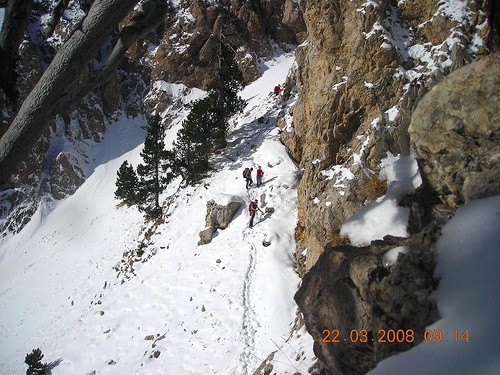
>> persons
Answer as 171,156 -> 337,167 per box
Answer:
274,84 -> 282,98
245,167 -> 253,189
248,199 -> 258,228
256,165 -> 263,187
